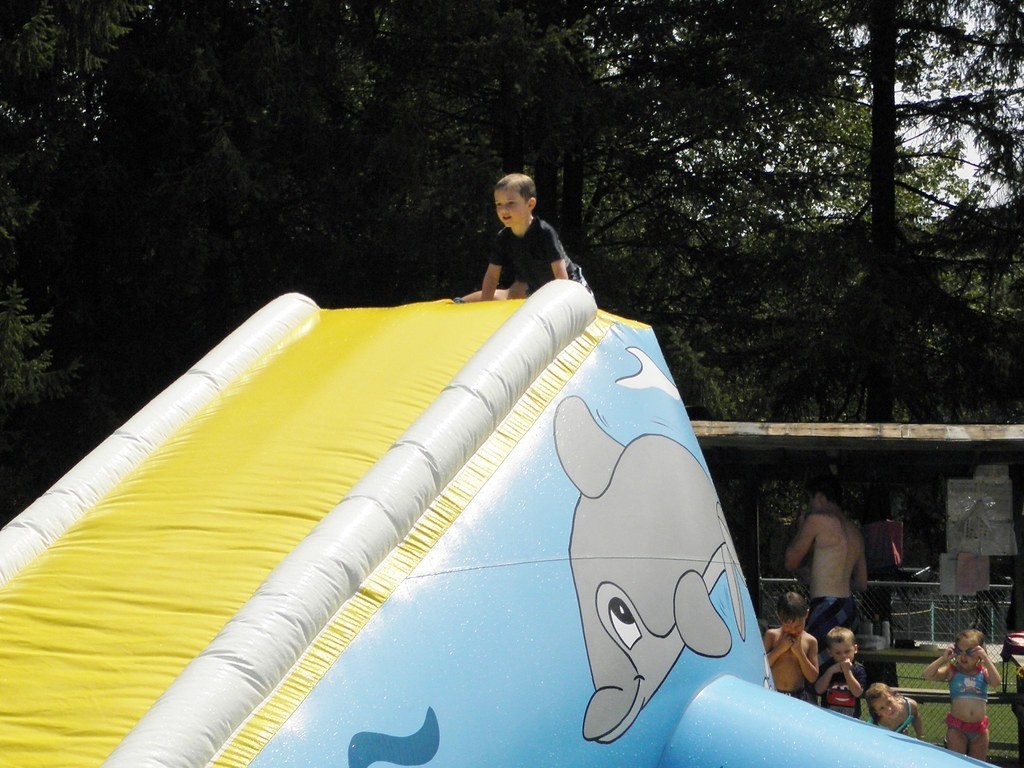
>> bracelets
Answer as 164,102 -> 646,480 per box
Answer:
917,735 -> 925,739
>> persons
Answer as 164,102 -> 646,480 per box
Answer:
803,624 -> 867,720
924,628 -> 1003,761
455,173 -> 594,303
864,683 -> 927,742
785,474 -> 867,655
762,591 -> 819,697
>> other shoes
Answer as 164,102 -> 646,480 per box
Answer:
453,297 -> 466,303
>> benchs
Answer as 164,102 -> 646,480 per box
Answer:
891,688 -> 1024,719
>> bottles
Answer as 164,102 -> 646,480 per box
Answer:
859,611 -> 891,651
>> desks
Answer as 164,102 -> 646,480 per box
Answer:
860,651 -> 947,666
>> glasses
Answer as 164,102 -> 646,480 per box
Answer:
954,645 -> 975,657
